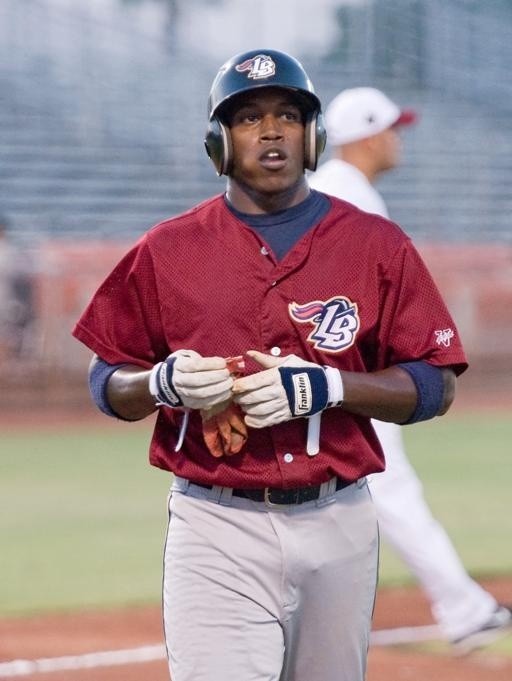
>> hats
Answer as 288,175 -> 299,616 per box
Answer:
324,85 -> 416,144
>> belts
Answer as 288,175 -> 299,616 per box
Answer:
189,476 -> 352,505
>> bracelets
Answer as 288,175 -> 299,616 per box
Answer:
323,364 -> 344,410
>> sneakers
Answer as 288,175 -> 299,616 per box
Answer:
449,603 -> 512,658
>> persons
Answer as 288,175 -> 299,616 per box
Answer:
69,45 -> 473,681
303,87 -> 511,652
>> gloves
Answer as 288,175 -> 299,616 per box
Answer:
149,348 -> 235,410
231,349 -> 344,428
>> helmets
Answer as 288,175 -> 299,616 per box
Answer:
204,47 -> 326,176
